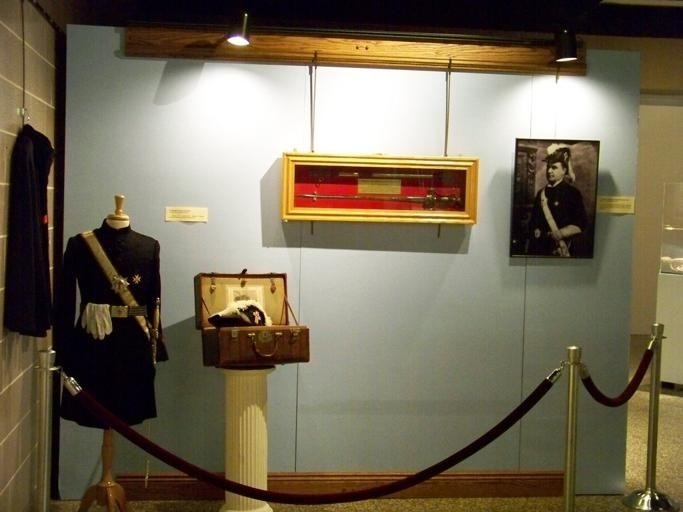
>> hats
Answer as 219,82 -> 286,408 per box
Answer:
541,147 -> 570,167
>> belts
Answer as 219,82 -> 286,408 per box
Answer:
79,302 -> 148,318
530,228 -> 552,240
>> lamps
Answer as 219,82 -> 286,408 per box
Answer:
226,11 -> 252,48
552,25 -> 579,65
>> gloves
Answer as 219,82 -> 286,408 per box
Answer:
80,302 -> 113,340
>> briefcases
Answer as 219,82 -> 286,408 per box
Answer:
195,269 -> 310,368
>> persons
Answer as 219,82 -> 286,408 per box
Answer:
521,144 -> 594,257
47,211 -> 168,429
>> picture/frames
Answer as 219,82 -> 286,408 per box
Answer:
509,136 -> 600,260
